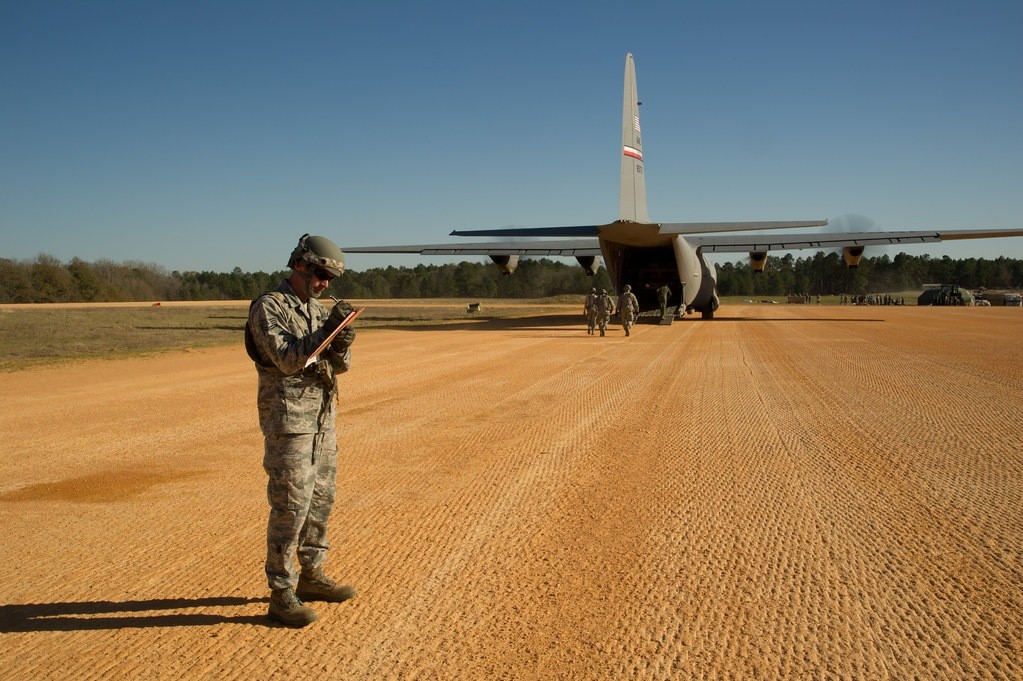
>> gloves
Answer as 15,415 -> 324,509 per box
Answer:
324,301 -> 353,333
333,325 -> 356,351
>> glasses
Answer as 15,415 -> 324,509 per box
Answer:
302,261 -> 333,282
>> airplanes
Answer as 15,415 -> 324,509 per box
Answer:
335,54 -> 1023,326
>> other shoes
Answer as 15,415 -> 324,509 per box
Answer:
600,328 -> 605,336
592,332 -> 594,334
626,327 -> 629,335
588,329 -> 590,334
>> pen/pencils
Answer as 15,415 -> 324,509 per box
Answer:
329,295 -> 338,303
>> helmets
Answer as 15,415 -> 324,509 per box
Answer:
599,289 -> 607,294
589,287 -> 596,293
623,285 -> 632,291
287,233 -> 345,278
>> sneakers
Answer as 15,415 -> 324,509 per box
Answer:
268,589 -> 319,626
294,567 -> 357,602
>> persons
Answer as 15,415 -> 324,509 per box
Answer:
245,234 -> 355,624
583,287 -> 598,335
788,293 -> 822,304
851,294 -> 904,305
593,288 -> 616,337
616,285 -> 639,337
656,284 -> 672,320
841,294 -> 847,305
